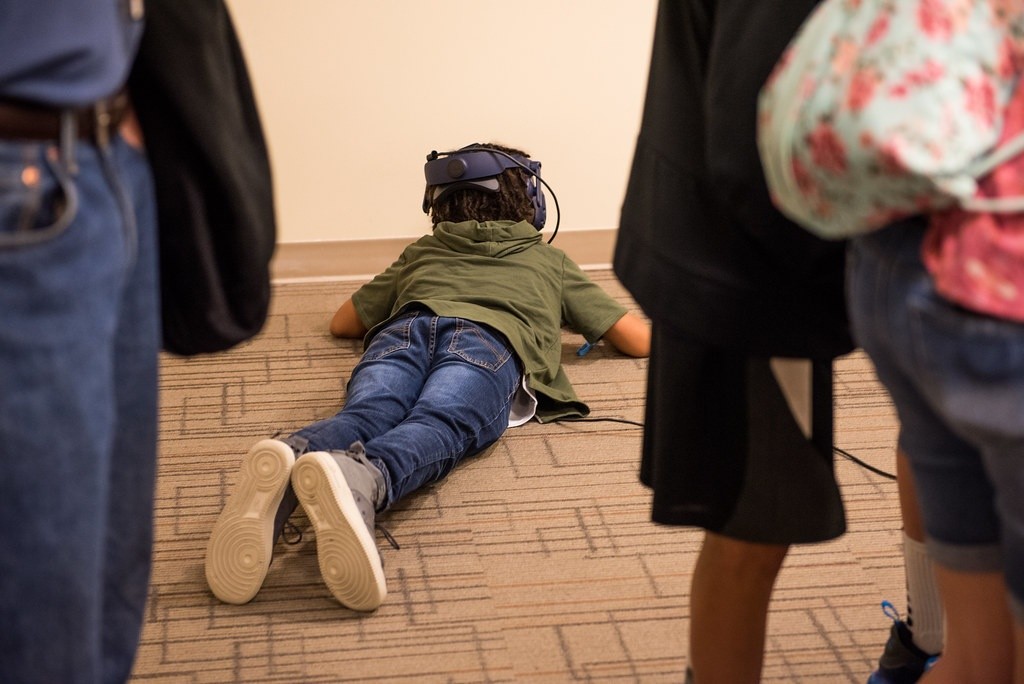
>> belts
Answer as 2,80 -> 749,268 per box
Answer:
0,92 -> 131,140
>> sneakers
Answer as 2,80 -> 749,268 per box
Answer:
204,435 -> 303,604
290,441 -> 400,611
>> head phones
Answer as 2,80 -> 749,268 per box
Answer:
422,151 -> 546,232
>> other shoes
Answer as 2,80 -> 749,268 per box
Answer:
867,602 -> 942,684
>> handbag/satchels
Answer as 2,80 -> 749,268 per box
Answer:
120,0 -> 276,358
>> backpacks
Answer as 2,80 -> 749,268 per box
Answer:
756,1 -> 1024,240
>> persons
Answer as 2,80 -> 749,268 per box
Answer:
0,0 -> 165,684
205,142 -> 651,612
609,0 -> 1024,684
